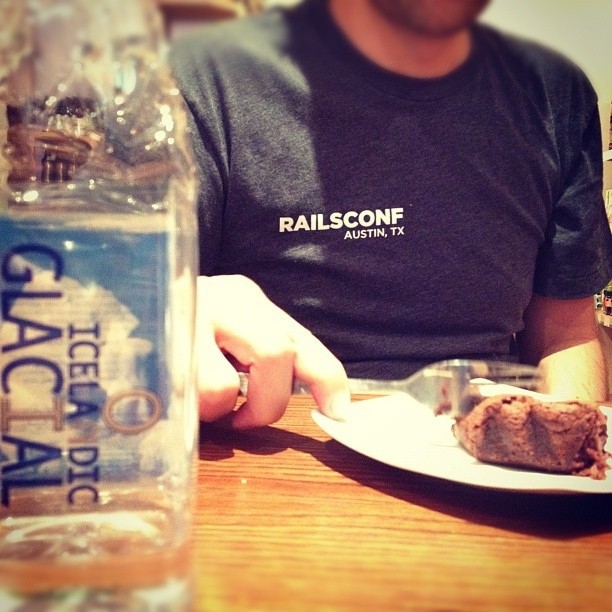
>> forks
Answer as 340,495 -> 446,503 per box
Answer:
231,361 -> 546,420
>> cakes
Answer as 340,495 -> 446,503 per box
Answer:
452,392 -> 611,479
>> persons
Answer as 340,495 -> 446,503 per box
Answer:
169,0 -> 611,438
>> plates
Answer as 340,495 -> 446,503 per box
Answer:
310,388 -> 612,502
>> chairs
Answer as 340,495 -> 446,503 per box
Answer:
157,1 -> 246,36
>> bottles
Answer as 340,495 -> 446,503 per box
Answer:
1,0 -> 199,610
